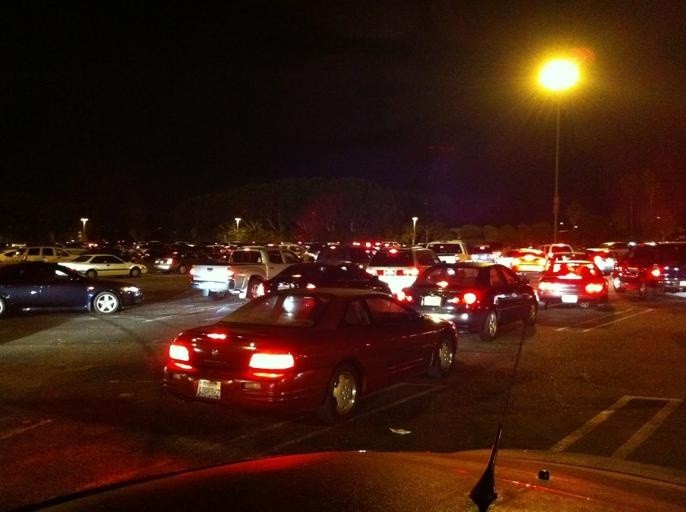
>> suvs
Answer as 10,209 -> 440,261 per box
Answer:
365,247 -> 442,293
612,243 -> 685,300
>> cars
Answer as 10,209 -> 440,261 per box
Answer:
152,243 -> 317,274
537,260 -> 607,307
159,287 -> 459,423
263,261 -> 392,299
57,254 -> 148,279
1,247 -> 87,262
396,263 -> 537,341
426,240 -> 629,275
0,262 -> 145,315
48,447 -> 686,507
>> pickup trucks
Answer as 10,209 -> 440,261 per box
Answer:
190,248 -> 301,301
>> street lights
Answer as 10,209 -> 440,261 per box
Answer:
232,216 -> 242,233
407,214 -> 419,238
530,49 -> 586,239
76,216 -> 89,238
